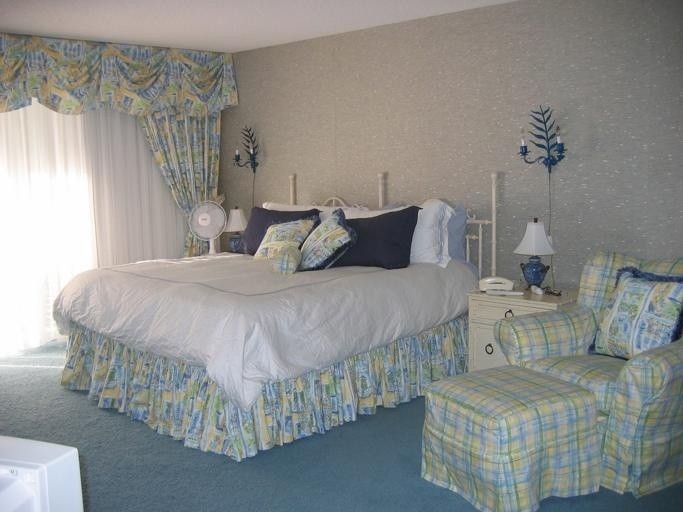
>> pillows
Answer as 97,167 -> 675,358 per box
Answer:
255,216 -> 320,257
588,266 -> 683,360
329,206 -> 423,269
243,206 -> 318,255
297,208 -> 357,272
359,199 -> 456,268
264,202 -> 358,222
256,242 -> 302,276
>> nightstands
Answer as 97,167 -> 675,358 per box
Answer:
468,291 -> 578,374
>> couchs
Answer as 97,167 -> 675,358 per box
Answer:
495,251 -> 683,499
421,365 -> 600,512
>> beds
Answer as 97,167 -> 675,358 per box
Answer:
52,171 -> 497,462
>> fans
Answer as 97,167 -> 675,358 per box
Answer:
189,200 -> 227,255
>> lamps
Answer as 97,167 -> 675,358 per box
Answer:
520,104 -> 565,246
232,126 -> 261,207
224,206 -> 248,252
512,217 -> 553,290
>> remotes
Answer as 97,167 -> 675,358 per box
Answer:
486,290 -> 524,296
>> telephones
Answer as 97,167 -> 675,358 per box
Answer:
479,277 -> 515,293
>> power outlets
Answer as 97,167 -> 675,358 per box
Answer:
548,236 -> 553,246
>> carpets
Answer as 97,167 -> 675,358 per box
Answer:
0,356 -> 683,512
